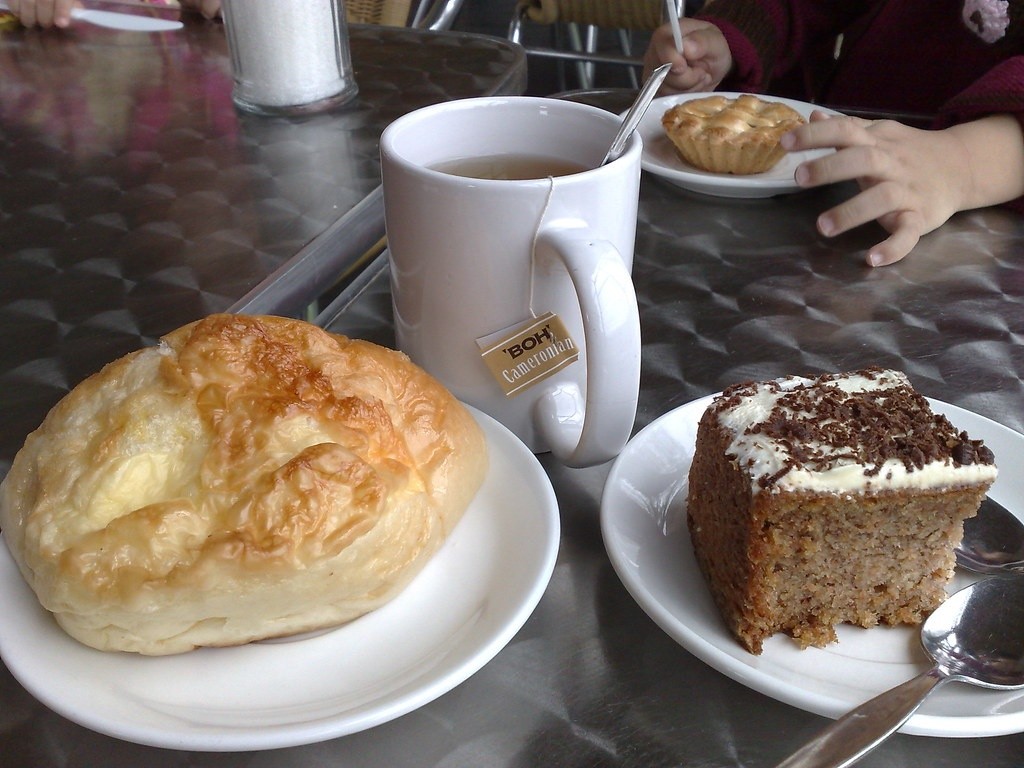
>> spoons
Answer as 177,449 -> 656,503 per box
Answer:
953,495 -> 1024,575
774,569 -> 1024,767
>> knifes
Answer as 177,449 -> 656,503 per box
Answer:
0,0 -> 183,31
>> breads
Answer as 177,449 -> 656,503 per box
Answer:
0,312 -> 487,656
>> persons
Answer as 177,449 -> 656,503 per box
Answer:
643,0 -> 1024,268
5,0 -> 223,26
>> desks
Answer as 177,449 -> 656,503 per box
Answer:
0,84 -> 1024,768
0,0 -> 528,482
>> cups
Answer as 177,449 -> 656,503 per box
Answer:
378,95 -> 643,468
221,0 -> 358,116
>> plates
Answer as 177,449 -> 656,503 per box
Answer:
0,402 -> 560,751
599,393 -> 1024,737
616,92 -> 846,198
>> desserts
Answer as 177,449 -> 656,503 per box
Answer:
662,94 -> 807,174
685,365 -> 1001,656
